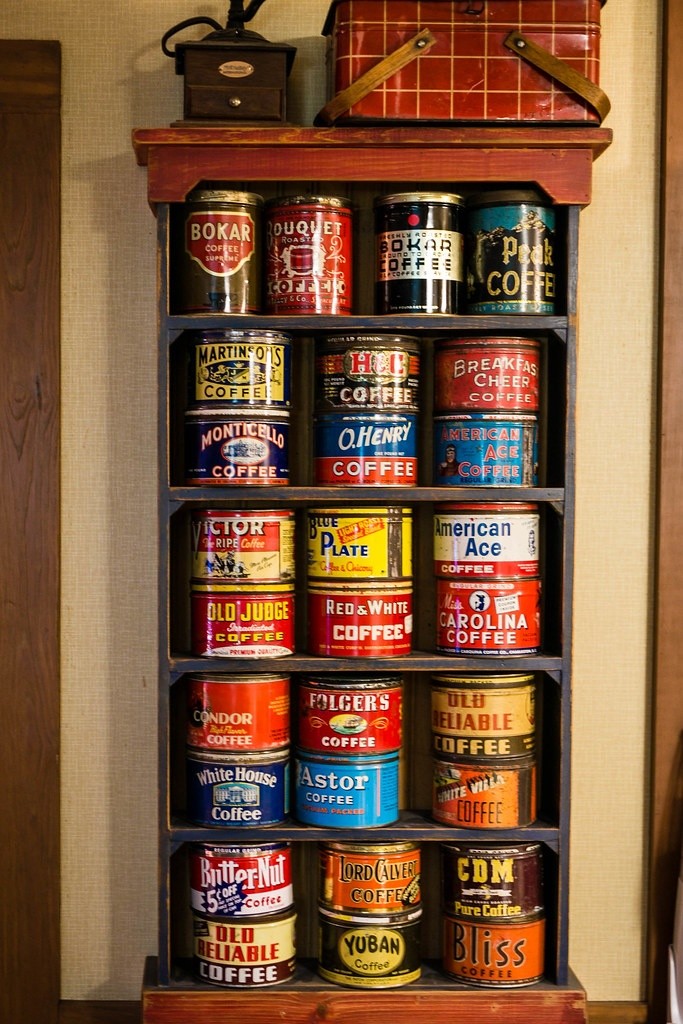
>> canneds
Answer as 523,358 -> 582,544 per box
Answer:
179,187 -> 555,991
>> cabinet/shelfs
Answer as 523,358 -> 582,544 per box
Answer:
132,127 -> 616,1024
